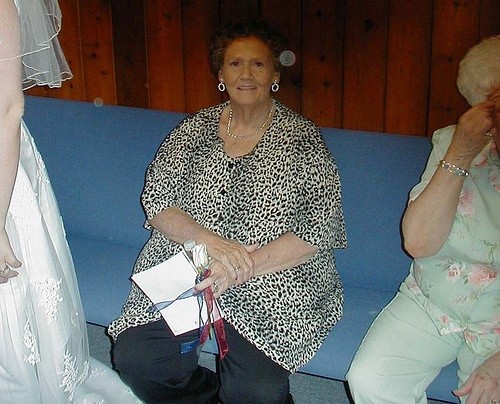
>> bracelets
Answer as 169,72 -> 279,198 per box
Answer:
438,160 -> 470,178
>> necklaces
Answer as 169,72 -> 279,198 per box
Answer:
226,95 -> 276,140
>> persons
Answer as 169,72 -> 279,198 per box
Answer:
344,34 -> 500,404
106,14 -> 350,404
0,0 -> 91,404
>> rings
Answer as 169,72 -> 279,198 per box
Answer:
212,282 -> 220,291
233,265 -> 241,273
0,264 -> 11,276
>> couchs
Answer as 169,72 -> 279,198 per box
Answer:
22,91 -> 464,403
